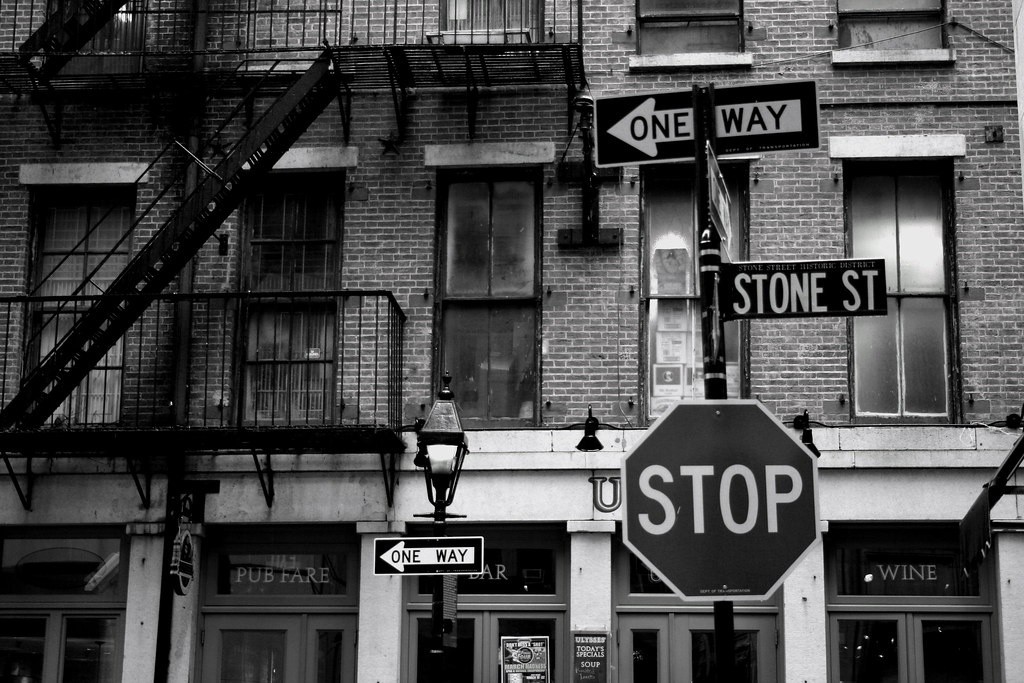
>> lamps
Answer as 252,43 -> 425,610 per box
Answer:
398,369 -> 473,524
577,406 -> 608,453
791,409 -> 820,459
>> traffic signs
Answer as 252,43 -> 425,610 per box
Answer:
592,77 -> 824,169
372,534 -> 487,577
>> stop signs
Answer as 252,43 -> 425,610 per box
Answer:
621,399 -> 821,603
